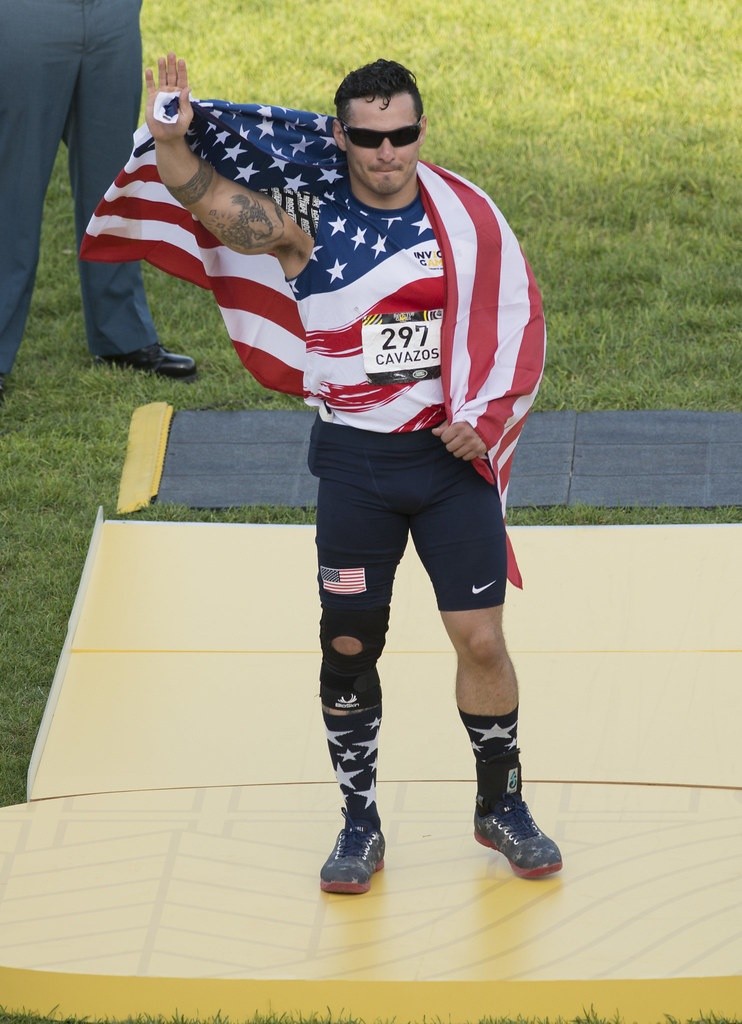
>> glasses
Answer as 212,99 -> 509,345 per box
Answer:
340,118 -> 422,150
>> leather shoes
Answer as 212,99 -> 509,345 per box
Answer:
95,343 -> 198,381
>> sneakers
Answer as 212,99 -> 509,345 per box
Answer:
320,816 -> 385,896
474,790 -> 564,881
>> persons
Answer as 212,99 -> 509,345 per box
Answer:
0,0 -> 199,407
145,51 -> 562,894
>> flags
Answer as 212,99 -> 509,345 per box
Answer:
72,86 -> 547,592
